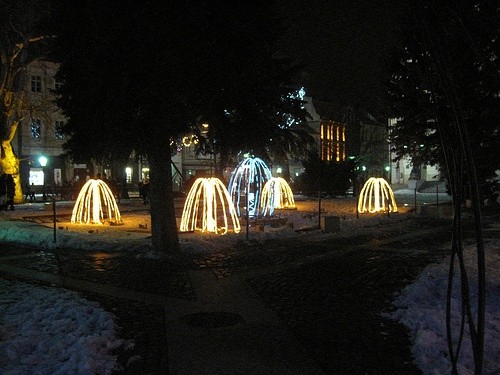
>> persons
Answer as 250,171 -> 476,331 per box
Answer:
0,172 -> 157,211
